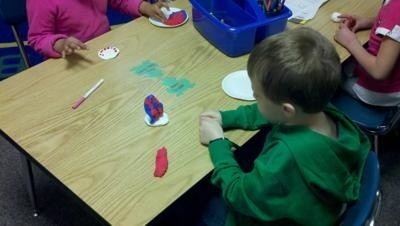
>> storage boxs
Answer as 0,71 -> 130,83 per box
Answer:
189,0 -> 292,57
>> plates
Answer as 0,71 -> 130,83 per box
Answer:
221,70 -> 257,101
148,7 -> 189,28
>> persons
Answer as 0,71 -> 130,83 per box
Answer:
334,0 -> 400,109
199,27 -> 370,226
25,0 -> 170,59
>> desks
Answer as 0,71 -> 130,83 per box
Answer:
0,0 -> 382,226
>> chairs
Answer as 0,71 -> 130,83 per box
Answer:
332,92 -> 400,158
202,151 -> 381,226
6,15 -> 43,69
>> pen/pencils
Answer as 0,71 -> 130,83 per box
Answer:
210,12 -> 224,23
72,78 -> 104,109
258,0 -> 286,17
